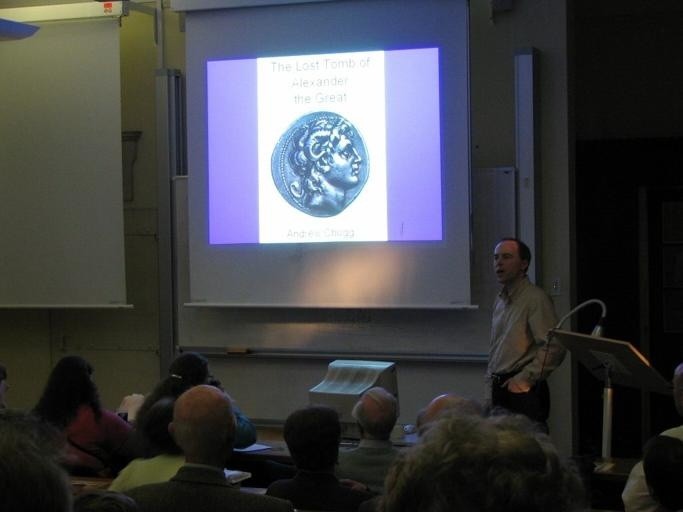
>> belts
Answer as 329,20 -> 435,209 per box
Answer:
490,375 -> 505,384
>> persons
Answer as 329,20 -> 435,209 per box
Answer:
118,385 -> 295,512
136,351 -> 258,451
360,398 -> 591,512
264,406 -> 380,512
333,386 -> 408,494
0,403 -> 74,512
71,486 -> 140,511
479,235 -> 565,437
103,395 -> 186,493
26,354 -> 143,477
638,431 -> 682,512
285,112 -> 365,216
413,390 -> 494,447
616,361 -> 682,512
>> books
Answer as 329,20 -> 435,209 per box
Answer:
69,478 -> 109,495
221,468 -> 252,485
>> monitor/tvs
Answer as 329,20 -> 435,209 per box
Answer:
308,359 -> 401,441
